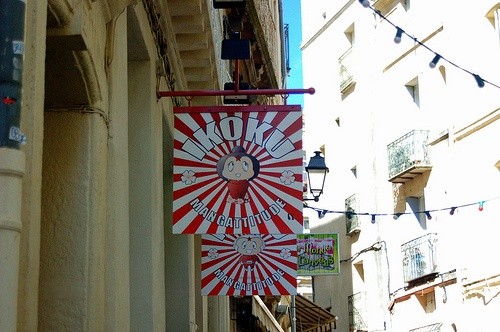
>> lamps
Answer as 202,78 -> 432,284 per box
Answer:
220,32 -> 250,59
303,151 -> 328,201
224,72 -> 248,103
